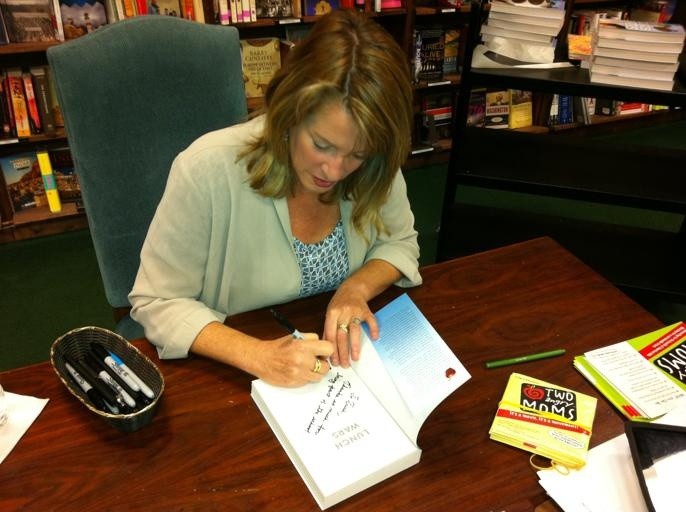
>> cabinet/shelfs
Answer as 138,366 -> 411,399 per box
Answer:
1,0 -> 435,243
435,0 -> 685,303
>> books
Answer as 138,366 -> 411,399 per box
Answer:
249,292 -> 472,511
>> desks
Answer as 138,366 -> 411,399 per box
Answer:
0,238 -> 683,512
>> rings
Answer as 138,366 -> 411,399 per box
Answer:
337,323 -> 348,333
313,360 -> 321,372
350,317 -> 361,325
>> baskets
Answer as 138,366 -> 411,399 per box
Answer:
50,325 -> 165,434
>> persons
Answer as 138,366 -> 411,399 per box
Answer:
127,10 -> 423,389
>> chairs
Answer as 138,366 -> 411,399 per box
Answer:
46,15 -> 243,323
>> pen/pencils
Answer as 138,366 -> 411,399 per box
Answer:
486,349 -> 567,369
268,306 -> 305,340
65,342 -> 156,415
